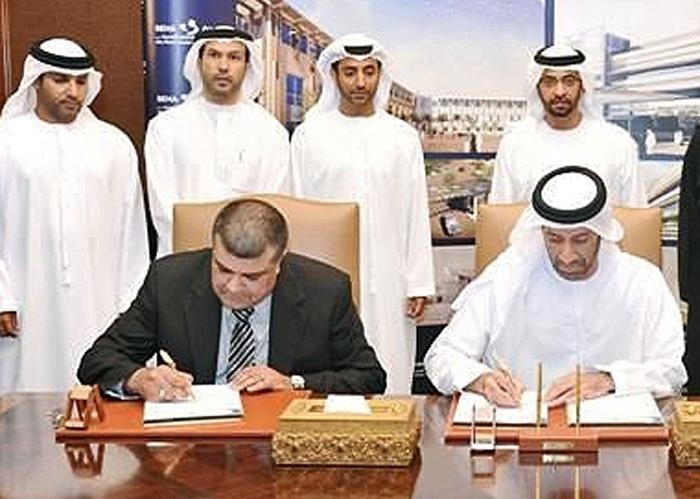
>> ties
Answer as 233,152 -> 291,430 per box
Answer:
224,306 -> 261,388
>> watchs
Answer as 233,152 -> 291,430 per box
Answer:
290,375 -> 306,390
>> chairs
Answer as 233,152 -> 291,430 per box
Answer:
172,195 -> 360,331
474,201 -> 661,291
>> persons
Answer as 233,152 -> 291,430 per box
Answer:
488,44 -> 650,210
0,35 -> 158,395
677,124 -> 700,396
76,199 -> 387,403
289,32 -> 436,395
423,166 -> 690,410
144,26 -> 295,260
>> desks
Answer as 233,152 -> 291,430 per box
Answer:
0,387 -> 700,498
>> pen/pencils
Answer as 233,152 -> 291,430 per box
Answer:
159,348 -> 196,400
489,353 -> 524,404
